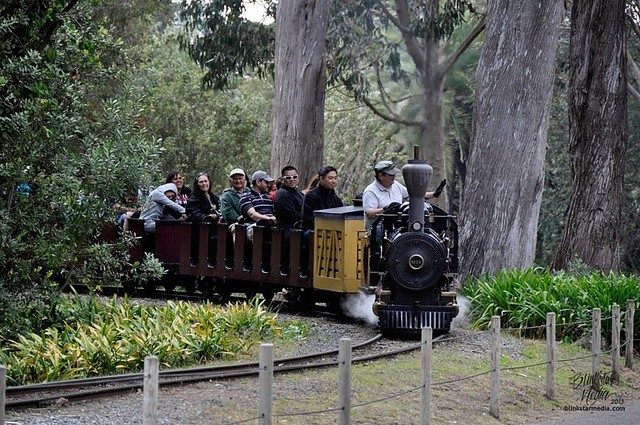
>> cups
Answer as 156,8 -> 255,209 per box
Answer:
282,175 -> 299,179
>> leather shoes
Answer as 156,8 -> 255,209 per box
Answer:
433,179 -> 446,197
383,202 -> 398,211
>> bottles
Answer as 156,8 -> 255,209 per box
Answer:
374,161 -> 401,175
230,168 -> 245,177
251,170 -> 275,182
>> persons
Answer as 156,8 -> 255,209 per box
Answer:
185,171 -> 221,240
164,169 -> 194,221
301,174 -> 321,195
301,166 -> 344,253
269,177 -> 283,201
239,171 -> 274,244
109,188 -> 143,233
363,161 -> 446,244
138,181 -> 189,240
274,166 -> 305,259
220,168 -> 252,254
